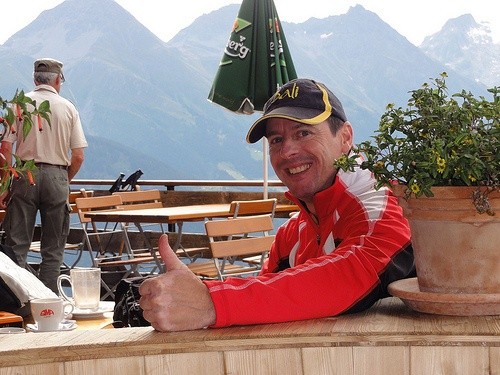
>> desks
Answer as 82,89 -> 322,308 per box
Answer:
84,203 -> 296,275
0,294 -> 500,375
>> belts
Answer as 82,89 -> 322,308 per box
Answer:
35,162 -> 66,168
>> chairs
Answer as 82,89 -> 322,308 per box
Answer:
186,197 -> 277,277
75,195 -> 163,280
28,190 -> 94,270
204,215 -> 276,282
112,188 -> 210,273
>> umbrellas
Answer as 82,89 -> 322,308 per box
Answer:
206,0 -> 297,200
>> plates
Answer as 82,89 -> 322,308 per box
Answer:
26,323 -> 79,332
64,306 -> 114,318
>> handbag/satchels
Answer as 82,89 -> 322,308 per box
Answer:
112,274 -> 159,329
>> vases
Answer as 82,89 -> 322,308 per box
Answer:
385,183 -> 500,316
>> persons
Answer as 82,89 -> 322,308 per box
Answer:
139,78 -> 416,332
0,58 -> 85,297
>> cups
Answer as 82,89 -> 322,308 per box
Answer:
57,268 -> 101,309
0,327 -> 25,334
29,298 -> 74,328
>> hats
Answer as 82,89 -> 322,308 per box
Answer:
245,78 -> 348,144
34,59 -> 65,82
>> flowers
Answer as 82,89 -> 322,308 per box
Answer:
332,68 -> 500,216
0,87 -> 53,198
